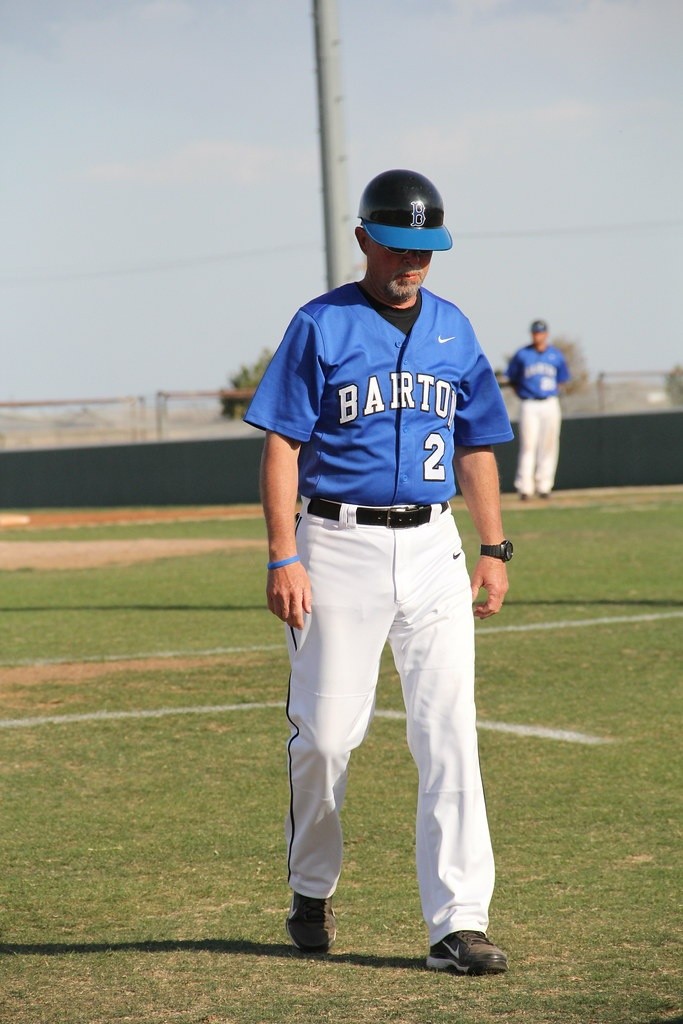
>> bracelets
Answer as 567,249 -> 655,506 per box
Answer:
267,554 -> 300,569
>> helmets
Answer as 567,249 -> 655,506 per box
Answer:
357,169 -> 452,252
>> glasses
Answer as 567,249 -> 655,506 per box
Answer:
384,244 -> 433,255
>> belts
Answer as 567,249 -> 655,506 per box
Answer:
521,395 -> 547,401
308,498 -> 448,530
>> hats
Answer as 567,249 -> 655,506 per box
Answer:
532,319 -> 547,332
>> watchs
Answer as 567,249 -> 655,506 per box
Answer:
480,539 -> 513,561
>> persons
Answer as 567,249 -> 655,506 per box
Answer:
505,321 -> 570,500
244,170 -> 515,977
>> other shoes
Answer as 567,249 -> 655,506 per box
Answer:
539,493 -> 549,499
520,493 -> 528,500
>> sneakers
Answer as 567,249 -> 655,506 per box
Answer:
286,885 -> 337,952
425,928 -> 508,974
